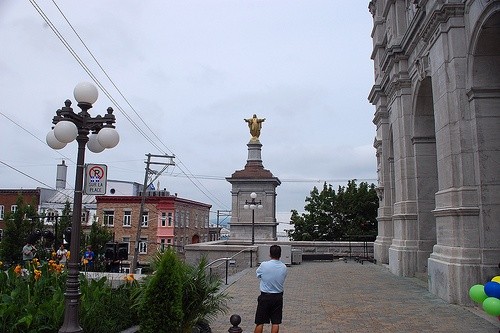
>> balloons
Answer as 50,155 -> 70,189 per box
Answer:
469,284 -> 488,303
482,297 -> 500,316
484,282 -> 500,300
491,276 -> 500,283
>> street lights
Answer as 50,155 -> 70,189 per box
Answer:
244,192 -> 263,268
44,81 -> 119,333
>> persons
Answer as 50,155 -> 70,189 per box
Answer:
103,248 -> 119,272
81,245 -> 94,271
22,241 -> 37,261
254,245 -> 287,333
57,244 -> 67,265
244,114 -> 266,140
